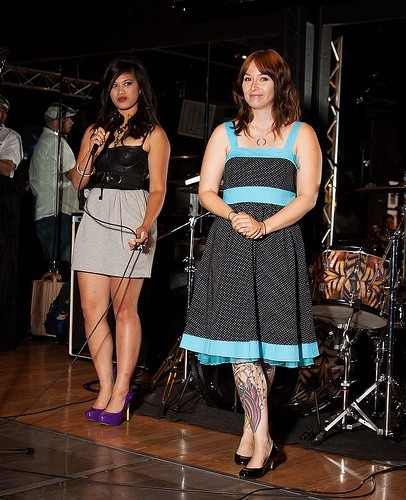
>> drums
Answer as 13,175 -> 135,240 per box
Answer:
190,319 -> 351,416
311,248 -> 391,329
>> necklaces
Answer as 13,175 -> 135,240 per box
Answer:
247,121 -> 277,146
112,115 -> 132,149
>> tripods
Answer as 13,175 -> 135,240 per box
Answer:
307,202 -> 406,447
144,211 -> 211,417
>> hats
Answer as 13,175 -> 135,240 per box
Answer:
44,102 -> 76,119
0,95 -> 10,112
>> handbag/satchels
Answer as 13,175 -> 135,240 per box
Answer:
31,272 -> 65,337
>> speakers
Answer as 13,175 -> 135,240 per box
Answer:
68,212 -> 119,366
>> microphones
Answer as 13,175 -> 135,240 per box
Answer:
91,110 -> 121,155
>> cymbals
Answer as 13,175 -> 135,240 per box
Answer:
174,235 -> 206,245
353,184 -> 406,191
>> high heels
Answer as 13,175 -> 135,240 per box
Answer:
235,451 -> 252,466
99,388 -> 133,427
239,439 -> 280,479
85,394 -> 111,422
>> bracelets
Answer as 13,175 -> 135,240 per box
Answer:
261,220 -> 267,236
76,163 -> 96,176
227,211 -> 236,222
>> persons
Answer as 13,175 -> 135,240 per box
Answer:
27,104 -> 90,341
196,48 -> 325,479
73,54 -> 172,427
0,95 -> 24,180
